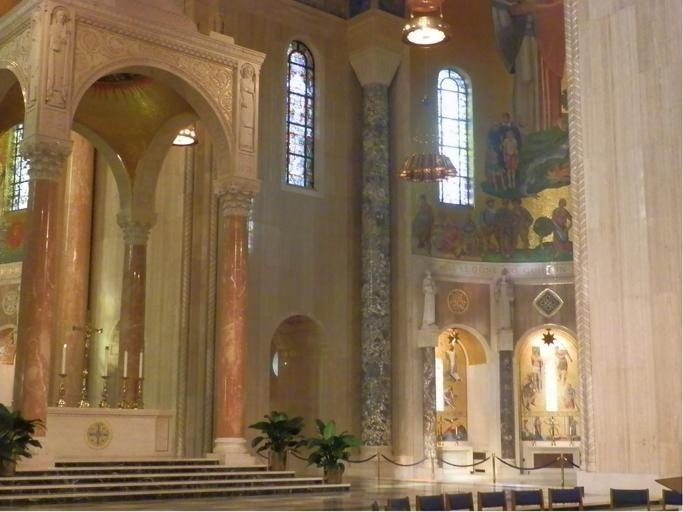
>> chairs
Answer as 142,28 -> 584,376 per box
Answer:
370,487 -> 682,510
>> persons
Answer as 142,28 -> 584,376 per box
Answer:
486,143 -> 507,199
495,199 -> 516,259
552,196 -> 572,260
480,194 -> 497,257
413,191 -> 432,258
503,131 -> 519,190
488,109 -> 524,165
510,199 -> 534,251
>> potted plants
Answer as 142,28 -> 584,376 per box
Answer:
1,404 -> 49,477
246,411 -> 364,485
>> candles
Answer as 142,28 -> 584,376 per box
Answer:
61,344 -> 67,375
104,350 -> 109,376
123,351 -> 128,377
139,352 -> 143,377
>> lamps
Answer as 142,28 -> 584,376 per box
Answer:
172,124 -> 199,145
401,0 -> 453,48
397,48 -> 458,183
